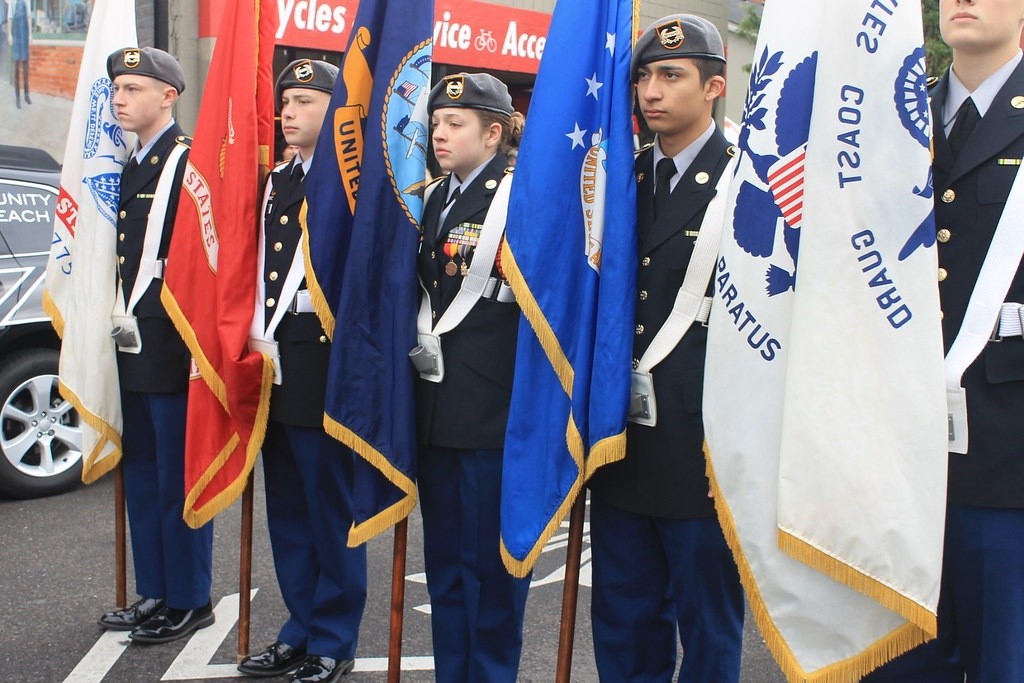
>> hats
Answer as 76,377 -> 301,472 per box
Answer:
630,14 -> 726,80
107,47 -> 185,95
427,72 -> 514,118
274,59 -> 340,115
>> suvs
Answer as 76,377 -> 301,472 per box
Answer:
0,145 -> 84,499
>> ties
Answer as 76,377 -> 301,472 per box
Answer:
290,163 -> 304,192
128,157 -> 137,176
948,97 -> 981,159
654,158 -> 678,213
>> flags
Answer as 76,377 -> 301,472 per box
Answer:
700,0 -> 950,683
301,0 -> 435,548
500,0 -> 641,578
42,0 -> 139,485
162,1 -> 276,530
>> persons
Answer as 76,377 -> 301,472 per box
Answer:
411,72 -> 533,683
585,13 -> 744,683
279,137 -> 300,163
98,45 -> 215,642
237,58 -> 367,683
858,0 -> 1024,683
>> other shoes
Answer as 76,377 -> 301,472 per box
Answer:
16,100 -> 21,109
24,97 -> 32,105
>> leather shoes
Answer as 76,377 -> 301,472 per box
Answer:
237,640 -> 309,676
128,596 -> 215,643
99,597 -> 166,631
289,655 -> 354,683
